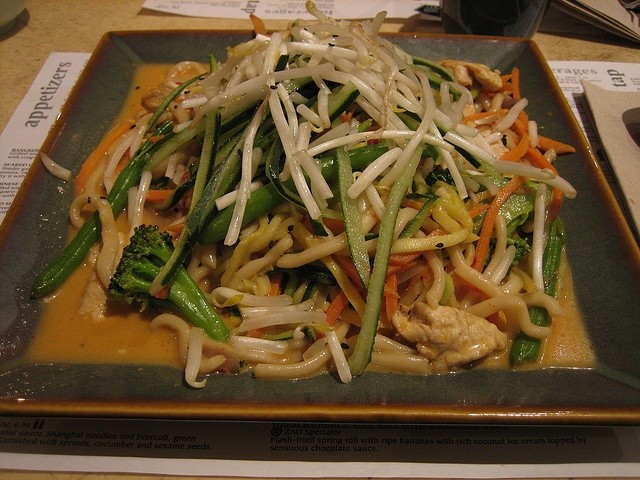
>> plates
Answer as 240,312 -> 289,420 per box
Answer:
0,28 -> 640,424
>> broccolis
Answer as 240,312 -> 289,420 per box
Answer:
107,223 -> 229,343
